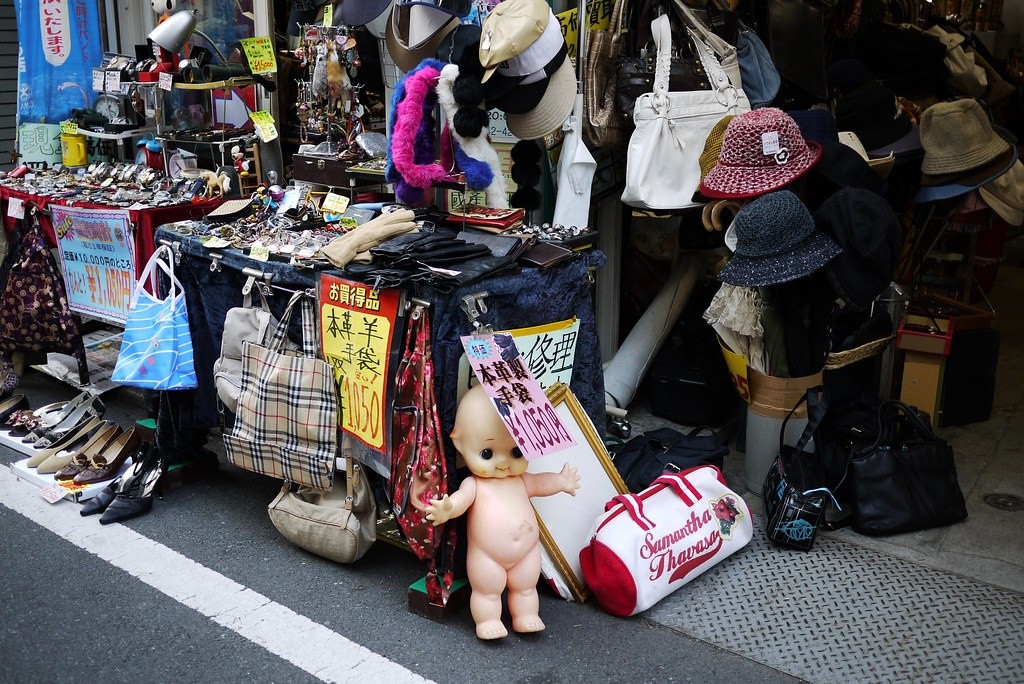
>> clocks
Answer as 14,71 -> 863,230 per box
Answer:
94,94 -> 125,119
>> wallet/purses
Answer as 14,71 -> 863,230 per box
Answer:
446,202 -> 537,262
519,241 -> 574,269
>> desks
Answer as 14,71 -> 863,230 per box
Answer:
0,185 -> 231,327
151,220 -> 607,623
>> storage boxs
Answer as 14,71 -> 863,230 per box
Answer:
895,312 -> 956,356
900,354 -> 945,436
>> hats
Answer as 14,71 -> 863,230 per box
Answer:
695,21 -> 1024,283
340,0 -> 575,139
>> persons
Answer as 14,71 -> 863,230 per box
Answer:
426,383 -> 581,639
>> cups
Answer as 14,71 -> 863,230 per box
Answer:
61,134 -> 87,166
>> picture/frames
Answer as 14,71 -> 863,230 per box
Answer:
530,381 -> 632,604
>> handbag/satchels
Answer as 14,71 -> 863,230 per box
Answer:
0,210 -> 85,354
576,422 -> 753,619
112,246 -> 199,389
759,372 -> 973,551
581,3 -> 783,211
267,465 -> 377,565
213,281 -> 349,491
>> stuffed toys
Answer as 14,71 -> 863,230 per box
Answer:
231,140 -> 251,176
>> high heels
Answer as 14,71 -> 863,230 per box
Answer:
0,365 -> 170,525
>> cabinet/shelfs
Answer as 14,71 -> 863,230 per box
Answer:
78,76 -> 257,166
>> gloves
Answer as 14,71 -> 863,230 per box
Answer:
320,207 -> 492,270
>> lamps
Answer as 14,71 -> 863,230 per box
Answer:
147,9 -> 227,61
